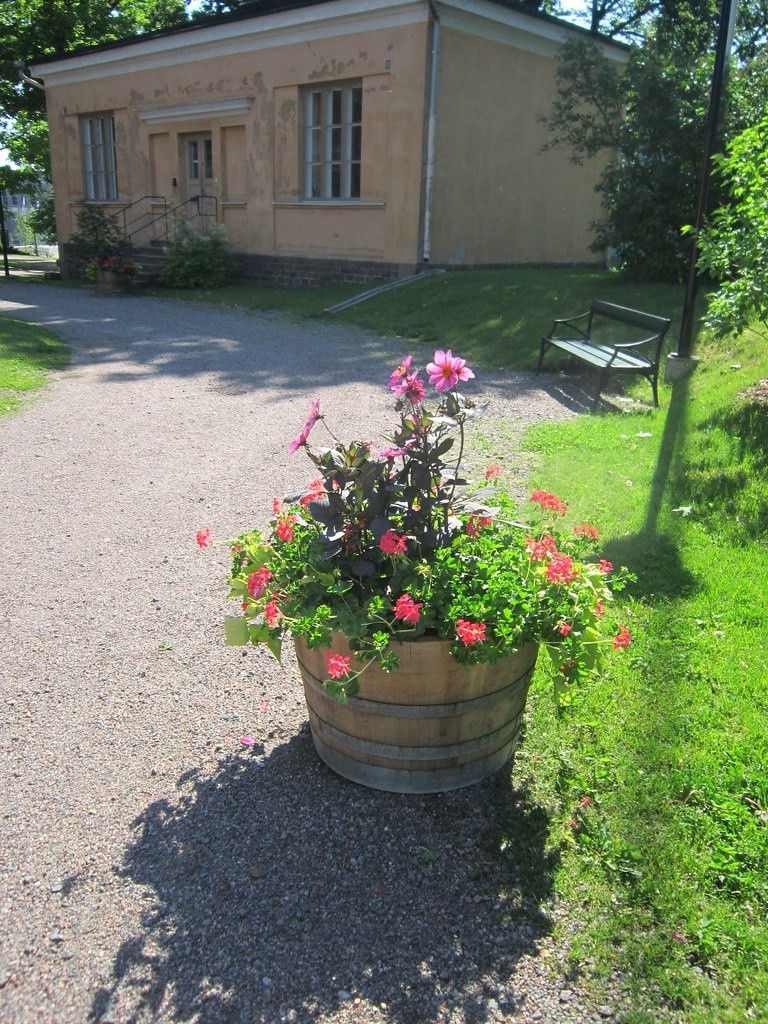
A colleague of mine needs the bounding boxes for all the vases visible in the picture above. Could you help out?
[285,511,549,793]
[95,270,131,295]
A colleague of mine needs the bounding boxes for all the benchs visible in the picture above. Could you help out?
[536,297,670,414]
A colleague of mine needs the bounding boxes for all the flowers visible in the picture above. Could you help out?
[86,234,139,283]
[197,351,638,707]
